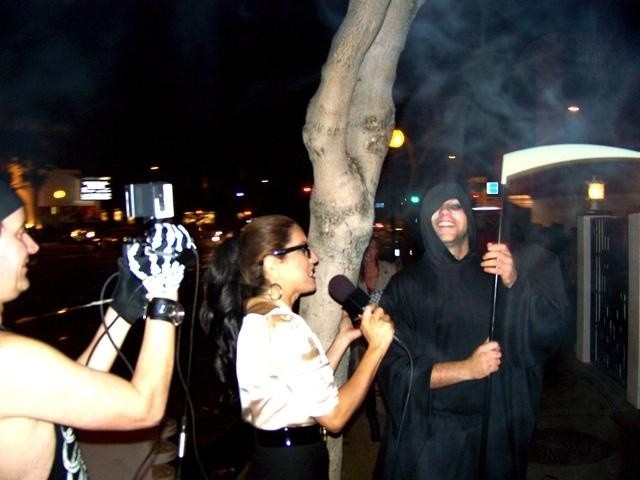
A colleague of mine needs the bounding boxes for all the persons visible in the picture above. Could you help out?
[371,182,574,478]
[355,233,396,441]
[1,179,192,480]
[198,213,399,480]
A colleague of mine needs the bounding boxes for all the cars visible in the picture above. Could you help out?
[373,211,403,255]
[194,208,262,271]
[69,223,131,251]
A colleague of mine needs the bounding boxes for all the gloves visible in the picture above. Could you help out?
[113,223,192,324]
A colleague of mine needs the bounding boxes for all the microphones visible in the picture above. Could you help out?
[329,276,405,351]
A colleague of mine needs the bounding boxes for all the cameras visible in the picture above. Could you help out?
[122,181,180,293]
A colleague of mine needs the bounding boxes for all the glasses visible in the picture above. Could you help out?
[274,242,312,259]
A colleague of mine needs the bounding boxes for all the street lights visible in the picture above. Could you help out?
[388,129,405,262]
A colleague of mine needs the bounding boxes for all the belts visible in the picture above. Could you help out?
[258,422,329,447]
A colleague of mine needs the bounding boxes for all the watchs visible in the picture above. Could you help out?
[141,297,187,326]
